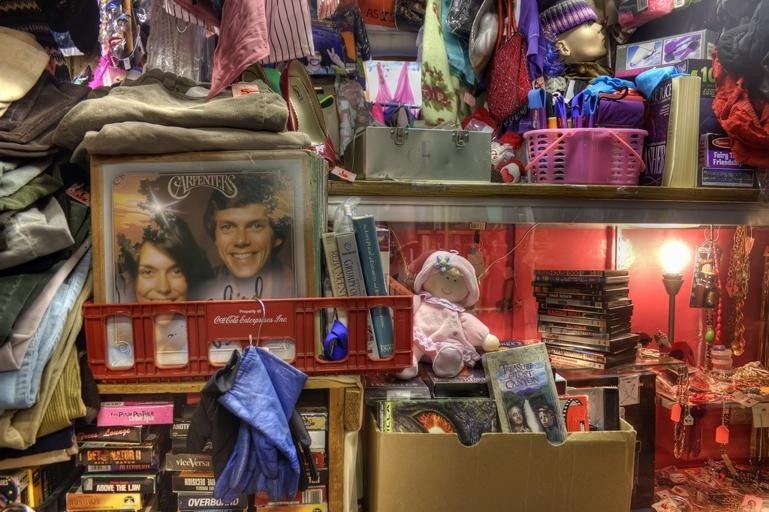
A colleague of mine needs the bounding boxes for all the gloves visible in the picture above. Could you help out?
[289,408,319,493]
[211,345,309,506]
[186,349,241,483]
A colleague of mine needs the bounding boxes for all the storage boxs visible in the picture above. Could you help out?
[368,401,636,512]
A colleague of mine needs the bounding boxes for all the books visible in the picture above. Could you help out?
[531,270,640,369]
[480,340,568,444]
[319,216,393,364]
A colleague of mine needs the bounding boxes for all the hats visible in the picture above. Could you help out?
[536,0,599,37]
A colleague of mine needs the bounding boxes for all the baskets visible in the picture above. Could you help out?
[522,126,651,186]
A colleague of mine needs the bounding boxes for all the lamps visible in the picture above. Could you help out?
[658,238,696,346]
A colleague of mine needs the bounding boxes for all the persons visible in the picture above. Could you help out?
[540,1,612,83]
[389,251,499,379]
[507,403,560,445]
[118,174,296,301]
